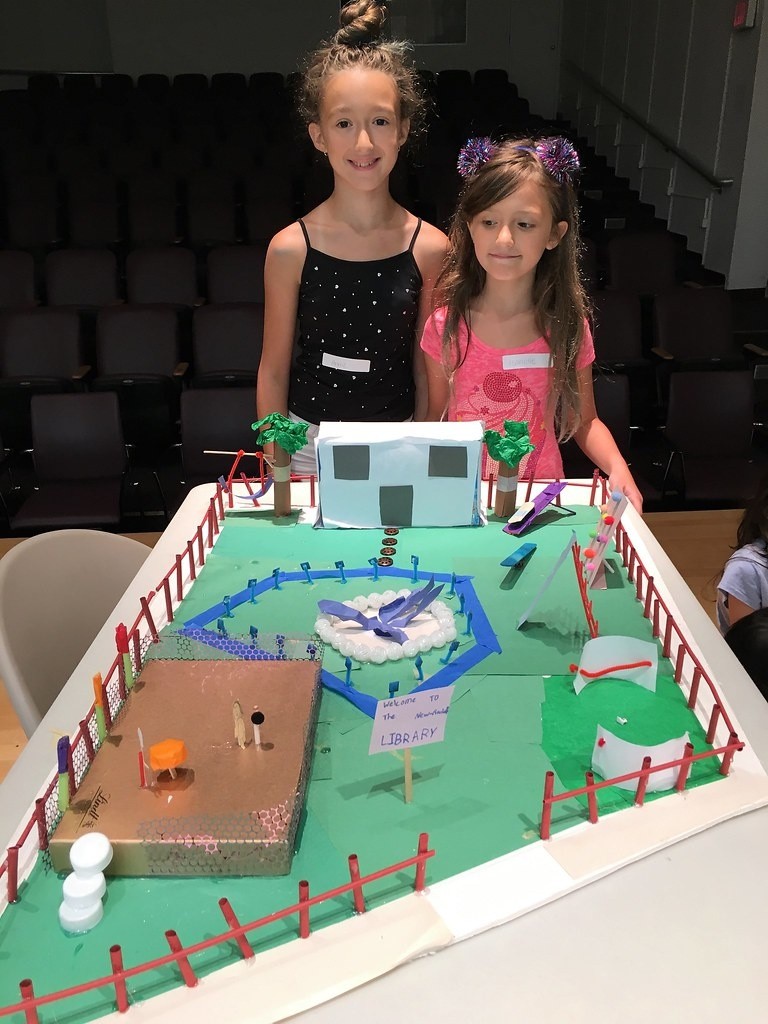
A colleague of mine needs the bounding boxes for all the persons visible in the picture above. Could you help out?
[255,0,451,484]
[420,136,643,516]
[702,483,768,624]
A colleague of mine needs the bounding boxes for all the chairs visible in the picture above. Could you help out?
[657,371,768,500]
[561,374,663,503]
[0,529,153,741]
[153,386,263,522]
[0,391,144,530]
[0,69,768,429]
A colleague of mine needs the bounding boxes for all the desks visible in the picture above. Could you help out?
[0,482,767,1024]
